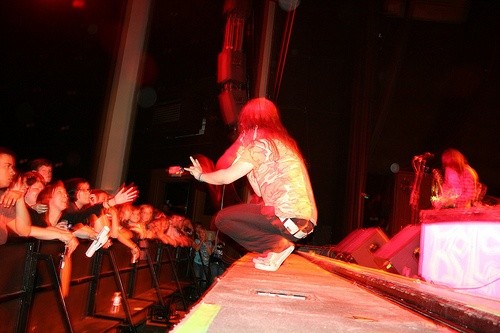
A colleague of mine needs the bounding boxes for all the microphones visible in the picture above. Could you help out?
[168,166,191,177]
[414,152,431,160]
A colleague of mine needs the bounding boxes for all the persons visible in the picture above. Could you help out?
[183,98,317,270]
[0,148,227,333]
[432,149,478,209]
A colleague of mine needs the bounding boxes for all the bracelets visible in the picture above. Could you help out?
[107,200,111,207]
[199,173,203,181]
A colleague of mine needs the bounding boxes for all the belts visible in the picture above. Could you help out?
[278,216,313,239]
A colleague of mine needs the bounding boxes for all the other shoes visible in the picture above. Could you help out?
[253,245,295,271]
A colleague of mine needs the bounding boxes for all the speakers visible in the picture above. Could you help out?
[331,227,390,268]
[373,224,421,278]
